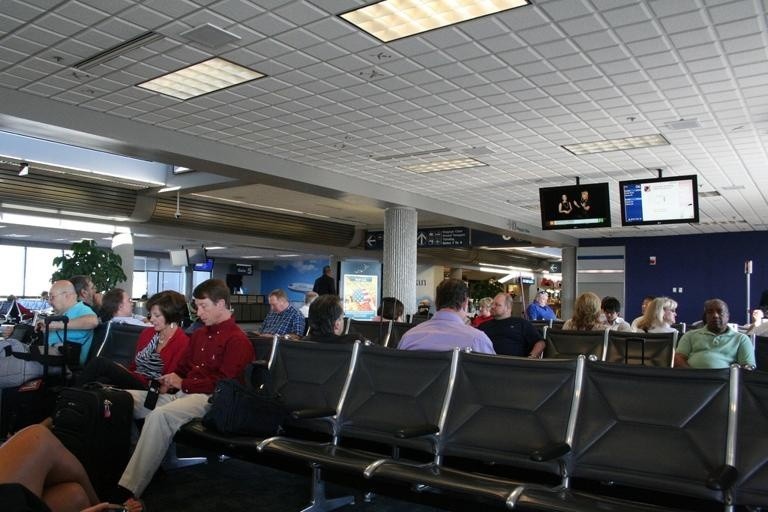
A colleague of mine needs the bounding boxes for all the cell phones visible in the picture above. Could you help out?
[144,376,179,411]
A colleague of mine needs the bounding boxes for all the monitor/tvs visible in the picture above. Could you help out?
[193,257,215,272]
[619,174,699,227]
[235,264,253,275]
[169,249,189,267]
[187,248,207,264]
[539,182,611,230]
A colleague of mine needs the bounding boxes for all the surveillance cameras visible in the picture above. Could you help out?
[175,212,182,219]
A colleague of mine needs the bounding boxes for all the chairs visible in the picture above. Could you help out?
[0,298,51,329]
[359,346,585,512]
[72,312,767,396]
[704,359,767,512]
[252,338,460,510]
[503,354,743,512]
[204,334,361,511]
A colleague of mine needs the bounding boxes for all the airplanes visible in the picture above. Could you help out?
[287,282,314,294]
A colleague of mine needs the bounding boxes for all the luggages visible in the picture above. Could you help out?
[3,315,134,502]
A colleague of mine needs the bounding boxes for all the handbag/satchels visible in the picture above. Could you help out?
[201,379,289,435]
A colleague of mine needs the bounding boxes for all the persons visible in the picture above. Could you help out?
[673,299,767,369]
[558,193,573,219]
[573,190,592,218]
[299,291,320,318]
[561,292,679,333]
[477,291,547,358]
[374,297,404,322]
[397,278,494,354]
[527,292,557,321]
[253,288,306,340]
[472,297,494,327]
[308,294,355,344]
[313,266,336,296]
[0,274,251,510]
